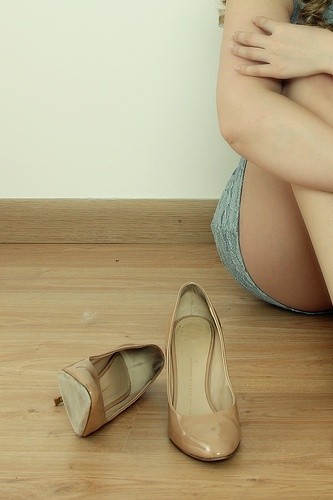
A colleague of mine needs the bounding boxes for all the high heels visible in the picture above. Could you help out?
[167,280,242,466]
[56,340,165,436]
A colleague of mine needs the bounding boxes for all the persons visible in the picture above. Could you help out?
[209,0,333,315]
